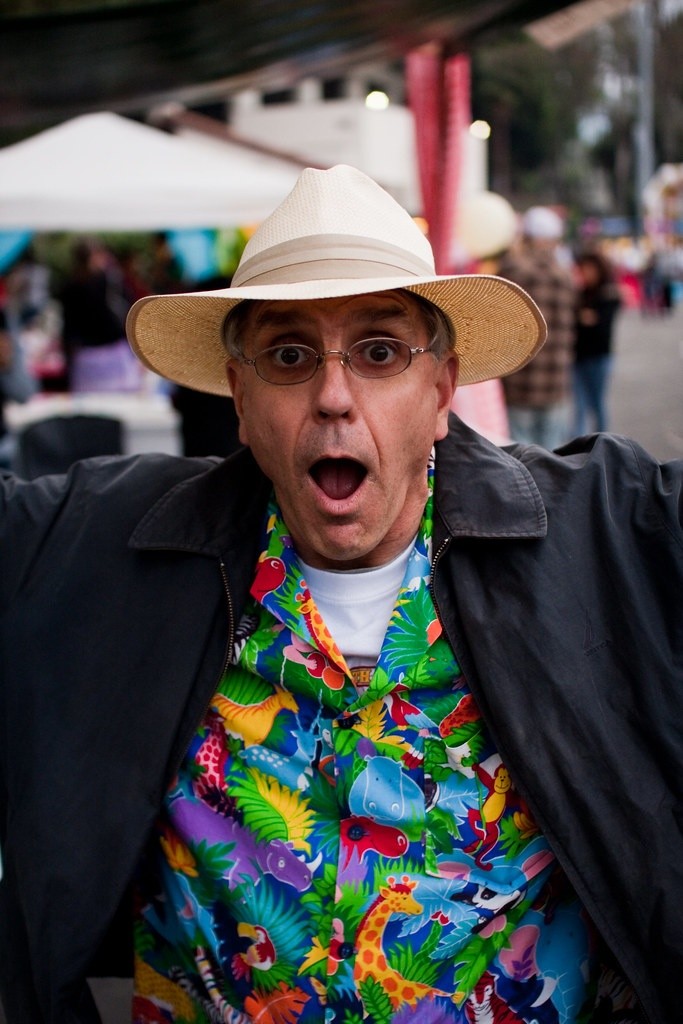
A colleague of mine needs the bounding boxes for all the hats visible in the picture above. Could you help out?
[524,205,564,240]
[125,165,547,398]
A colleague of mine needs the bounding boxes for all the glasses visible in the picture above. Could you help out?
[232,338,432,385]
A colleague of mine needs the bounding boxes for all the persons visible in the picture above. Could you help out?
[1,165,683,1023]
[0,206,683,447]
